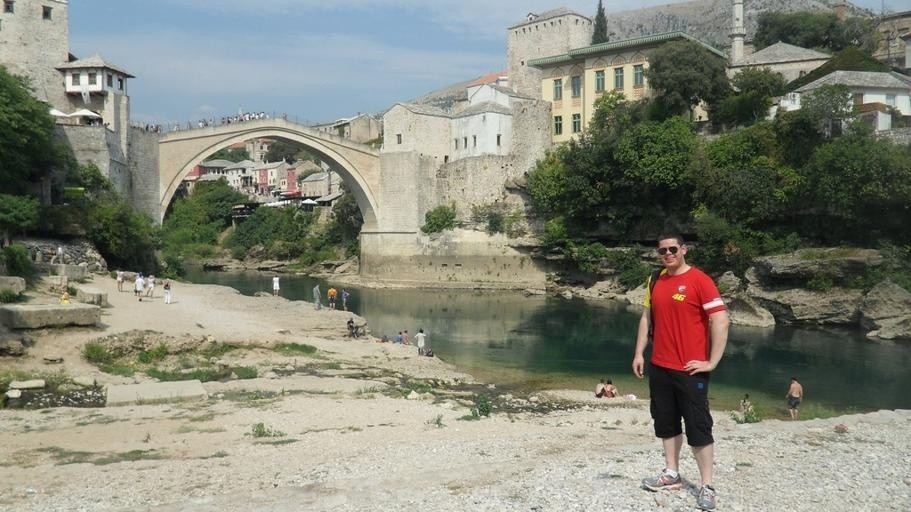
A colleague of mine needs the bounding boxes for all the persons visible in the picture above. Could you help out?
[785,376,804,421]
[146,274,157,298]
[401,329,411,346]
[116,268,124,292]
[394,331,402,344]
[413,327,427,355]
[738,393,752,414]
[271,273,280,297]
[56,243,65,265]
[101,111,287,134]
[162,277,174,304]
[327,285,337,311]
[312,284,322,311]
[631,232,730,511]
[341,288,350,311]
[347,317,358,338]
[133,271,143,297]
[58,292,72,305]
[135,275,145,302]
[603,379,616,398]
[593,378,606,398]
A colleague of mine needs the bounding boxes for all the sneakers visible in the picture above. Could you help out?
[642,470,682,491]
[696,485,716,511]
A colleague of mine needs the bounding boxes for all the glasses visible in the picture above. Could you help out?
[658,247,678,255]
